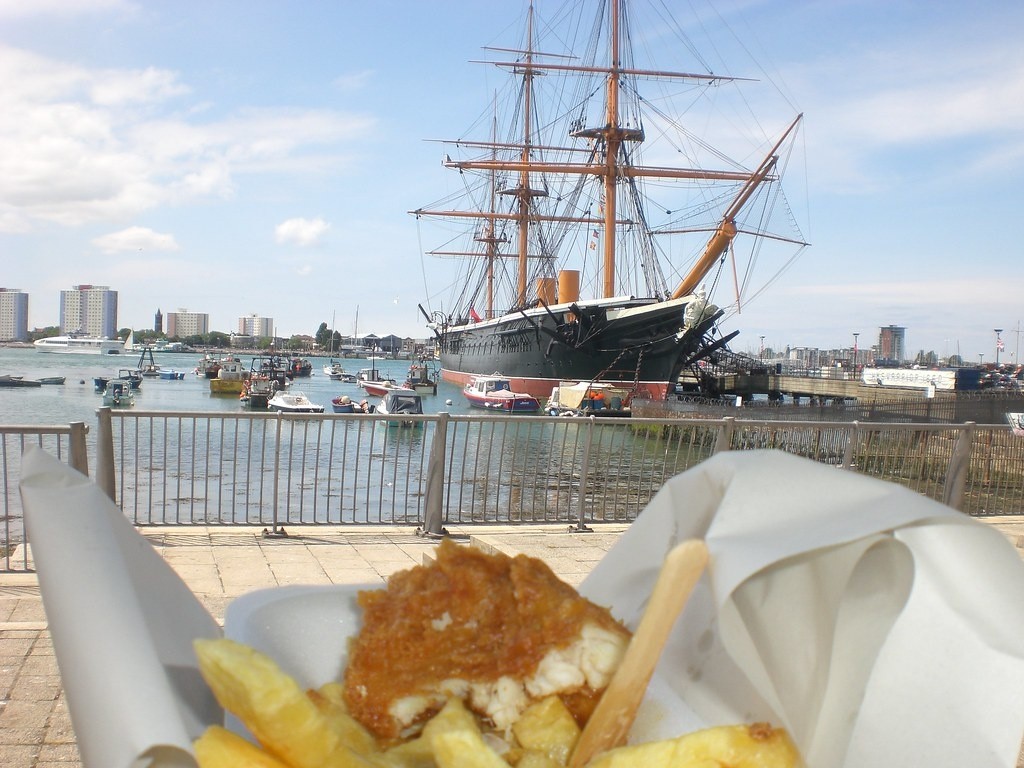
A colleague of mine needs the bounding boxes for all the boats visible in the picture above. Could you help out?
[402,364,437,396]
[194,351,312,408]
[102,379,136,408]
[544,381,632,417]
[359,379,415,398]
[0,375,42,388]
[376,388,424,428]
[267,391,325,414]
[35,376,66,384]
[333,396,375,414]
[463,371,542,412]
[34,334,126,355]
[94,342,185,390]
[329,371,359,384]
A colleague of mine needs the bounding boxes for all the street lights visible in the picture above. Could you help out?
[760,335,765,366]
[994,327,1005,371]
[978,352,985,366]
[853,331,859,380]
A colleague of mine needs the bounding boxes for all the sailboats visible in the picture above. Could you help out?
[405,0,812,416]
[354,341,397,387]
[324,309,347,375]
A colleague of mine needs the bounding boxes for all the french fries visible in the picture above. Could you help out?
[190,636,800,767]
